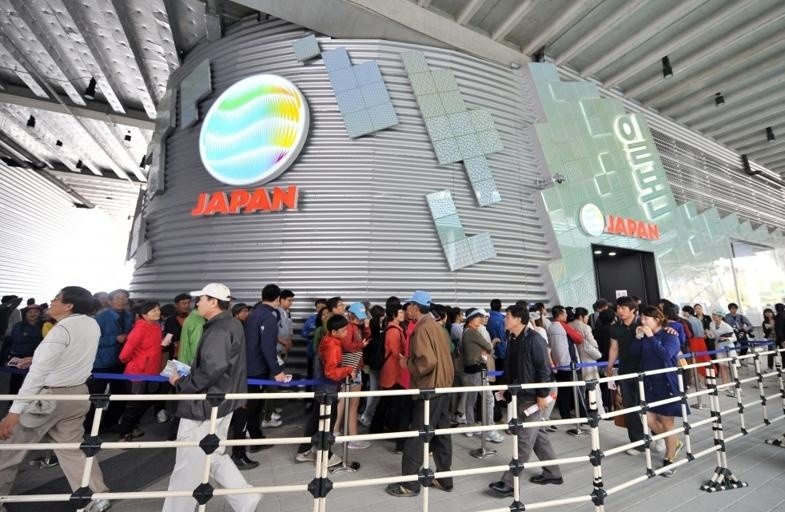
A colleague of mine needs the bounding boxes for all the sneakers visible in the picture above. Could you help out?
[85,498,110,512]
[429,478,452,492]
[261,419,282,427]
[485,430,503,442]
[662,460,676,477]
[333,433,344,444]
[271,412,281,419]
[157,410,168,423]
[296,450,317,462]
[655,437,667,452]
[465,430,481,437]
[238,493,263,512]
[132,429,144,437]
[348,440,371,450]
[120,435,130,450]
[386,483,419,497]
[670,439,684,459]
[327,454,343,467]
[626,448,640,455]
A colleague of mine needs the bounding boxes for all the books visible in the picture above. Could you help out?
[161,359,191,379]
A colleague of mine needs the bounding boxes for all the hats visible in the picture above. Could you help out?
[466,309,483,316]
[190,283,231,302]
[404,291,431,306]
[348,303,368,319]
[479,309,490,317]
[712,308,725,317]
[18,388,56,428]
[529,312,540,321]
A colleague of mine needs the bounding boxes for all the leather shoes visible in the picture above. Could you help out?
[530,476,563,485]
[488,480,514,496]
[249,445,273,453]
[231,454,259,470]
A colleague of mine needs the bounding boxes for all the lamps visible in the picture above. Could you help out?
[663,55,673,79]
[715,96,725,106]
[25,114,38,130]
[84,78,95,99]
[766,126,775,141]
[139,154,149,170]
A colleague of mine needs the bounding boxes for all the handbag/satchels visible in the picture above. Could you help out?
[338,352,364,371]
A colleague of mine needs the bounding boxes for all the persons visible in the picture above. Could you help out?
[165,293,192,421]
[296,314,356,467]
[359,305,386,427]
[393,320,417,454]
[490,306,563,494]
[386,297,400,307]
[693,304,721,378]
[39,303,57,324]
[548,305,586,417]
[657,299,667,311]
[595,300,617,410]
[0,295,23,350]
[313,306,333,353]
[477,309,507,423]
[629,305,691,467]
[449,306,468,424]
[128,298,143,323]
[90,299,101,317]
[775,303,785,373]
[41,309,57,337]
[178,297,208,367]
[21,299,37,321]
[94,292,110,315]
[371,303,405,433]
[516,301,558,431]
[300,299,327,392]
[230,284,285,470]
[163,284,264,512]
[724,303,755,367]
[462,308,506,444]
[7,305,43,407]
[704,310,741,398]
[486,300,508,408]
[119,302,163,449]
[663,303,695,393]
[607,296,680,456]
[83,289,134,433]
[333,302,372,450]
[588,302,602,336]
[763,309,779,373]
[232,302,249,320]
[430,302,452,331]
[386,291,455,497]
[682,306,717,378]
[158,304,174,423]
[0,287,111,512]
[328,297,346,314]
[260,289,294,427]
[566,308,607,414]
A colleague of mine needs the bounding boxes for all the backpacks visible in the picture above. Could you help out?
[314,354,338,402]
[363,325,402,370]
